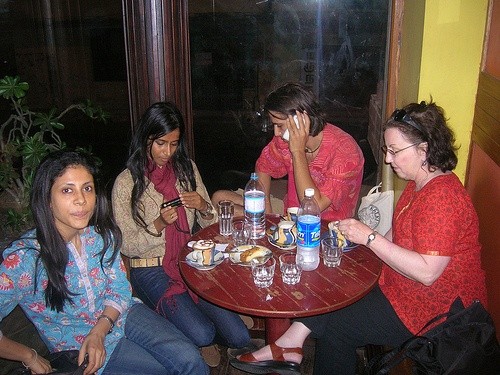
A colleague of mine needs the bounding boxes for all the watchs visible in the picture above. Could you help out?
[365,230,378,248]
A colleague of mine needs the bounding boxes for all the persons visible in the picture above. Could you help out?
[229,95,490,375]
[110,100,255,368]
[212,78,365,222]
[1,145,211,375]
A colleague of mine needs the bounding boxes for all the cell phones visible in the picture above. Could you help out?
[284,115,306,142]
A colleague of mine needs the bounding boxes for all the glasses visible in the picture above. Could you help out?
[381,141,422,155]
[390,109,422,138]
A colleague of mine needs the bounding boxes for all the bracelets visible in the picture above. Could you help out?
[144,225,163,238]
[97,314,115,335]
[200,201,212,217]
[21,348,38,369]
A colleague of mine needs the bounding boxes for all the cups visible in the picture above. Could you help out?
[321,237,344,267]
[287,207,302,225]
[327,220,354,248]
[279,251,304,285]
[230,220,252,246]
[217,200,234,237]
[251,256,276,288]
[192,245,215,266]
[277,220,297,245]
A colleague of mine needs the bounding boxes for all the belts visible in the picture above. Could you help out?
[129,257,163,268]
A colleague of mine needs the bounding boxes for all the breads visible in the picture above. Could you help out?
[240,246,266,262]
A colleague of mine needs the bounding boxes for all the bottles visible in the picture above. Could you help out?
[297,187,321,270]
[244,173,266,238]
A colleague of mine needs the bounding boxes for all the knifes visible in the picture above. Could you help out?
[221,250,244,253]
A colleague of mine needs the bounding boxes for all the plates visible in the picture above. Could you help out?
[230,245,272,265]
[321,231,359,252]
[185,250,224,270]
[280,216,287,221]
[267,235,298,251]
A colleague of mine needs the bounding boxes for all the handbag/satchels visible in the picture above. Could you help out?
[358,181,395,236]
[365,296,500,375]
[6,350,95,375]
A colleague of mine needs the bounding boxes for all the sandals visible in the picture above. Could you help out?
[230,342,304,375]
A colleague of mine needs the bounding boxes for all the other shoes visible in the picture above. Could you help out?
[201,344,221,368]
[239,314,254,330]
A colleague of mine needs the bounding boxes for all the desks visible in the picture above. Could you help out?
[178,215,382,347]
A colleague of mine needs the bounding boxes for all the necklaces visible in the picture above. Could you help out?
[304,139,324,154]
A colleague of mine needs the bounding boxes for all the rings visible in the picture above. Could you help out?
[171,214,174,219]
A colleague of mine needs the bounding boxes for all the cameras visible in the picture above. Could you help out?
[162,197,183,209]
